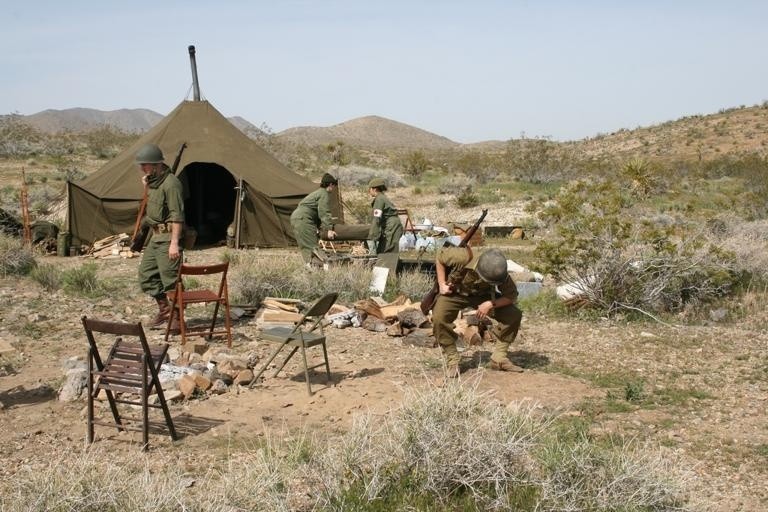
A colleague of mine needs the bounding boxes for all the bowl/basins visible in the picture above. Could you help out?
[413,224,433,230]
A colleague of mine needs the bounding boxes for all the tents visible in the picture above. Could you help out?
[67,100,344,257]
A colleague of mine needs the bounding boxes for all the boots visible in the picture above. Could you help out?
[490,339,524,373]
[442,343,463,378]
[169,307,186,335]
[145,293,171,327]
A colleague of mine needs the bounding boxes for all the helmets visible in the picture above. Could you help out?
[474,250,508,285]
[135,143,165,164]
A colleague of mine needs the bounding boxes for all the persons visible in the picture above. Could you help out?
[291,173,337,263]
[368,179,403,254]
[136,144,186,335]
[433,248,523,377]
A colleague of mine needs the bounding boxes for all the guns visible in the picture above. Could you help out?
[419,209,487,315]
[130,142,186,252]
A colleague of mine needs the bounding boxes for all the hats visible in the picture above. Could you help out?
[368,178,385,188]
[321,172,338,184]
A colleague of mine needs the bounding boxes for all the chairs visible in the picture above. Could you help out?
[81,314,179,452]
[247,292,340,397]
[394,209,422,241]
[162,260,233,348]
